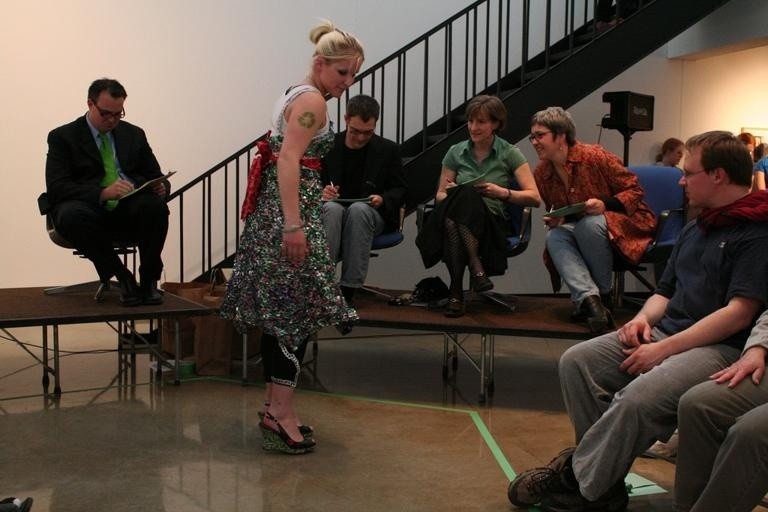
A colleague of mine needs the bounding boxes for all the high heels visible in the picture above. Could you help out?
[568,291,615,322]
[576,295,617,330]
[256,411,314,437]
[258,422,317,454]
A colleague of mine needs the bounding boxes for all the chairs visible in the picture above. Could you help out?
[416,174,531,312]
[38,193,137,302]
[568,166,687,323]
[336,205,405,305]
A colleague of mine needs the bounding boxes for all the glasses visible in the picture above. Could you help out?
[94,105,125,120]
[349,122,374,138]
[682,170,705,176]
[529,130,552,144]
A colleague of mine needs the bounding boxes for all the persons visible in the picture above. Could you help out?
[219,19,366,455]
[655,138,686,168]
[435,94,542,318]
[671,308,767,512]
[736,132,767,194]
[529,107,659,336]
[45,79,171,307]
[321,95,408,312]
[506,131,767,512]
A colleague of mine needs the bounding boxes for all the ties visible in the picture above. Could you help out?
[98,132,120,211]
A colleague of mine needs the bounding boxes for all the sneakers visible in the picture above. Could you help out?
[508,447,576,509]
[641,428,679,458]
[541,477,631,512]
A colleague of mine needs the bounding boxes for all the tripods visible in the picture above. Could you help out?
[612,136,657,304]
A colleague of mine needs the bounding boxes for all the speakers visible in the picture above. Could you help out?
[601,91,655,136]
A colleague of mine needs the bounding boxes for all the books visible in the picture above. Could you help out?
[331,197,371,205]
[118,170,177,200]
[543,202,586,217]
[446,173,487,195]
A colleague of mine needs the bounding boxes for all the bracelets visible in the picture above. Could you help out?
[505,189,513,202]
[281,225,301,234]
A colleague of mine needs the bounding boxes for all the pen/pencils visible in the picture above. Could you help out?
[330,181,340,197]
[447,179,452,183]
[118,171,127,180]
[544,204,554,232]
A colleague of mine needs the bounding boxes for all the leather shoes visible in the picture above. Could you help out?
[443,297,466,317]
[140,281,165,305]
[118,269,142,307]
[471,271,493,292]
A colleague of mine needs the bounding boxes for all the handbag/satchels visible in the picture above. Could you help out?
[413,276,448,302]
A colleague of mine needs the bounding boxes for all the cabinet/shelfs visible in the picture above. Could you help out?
[0,282,212,407]
[312,285,654,406]
